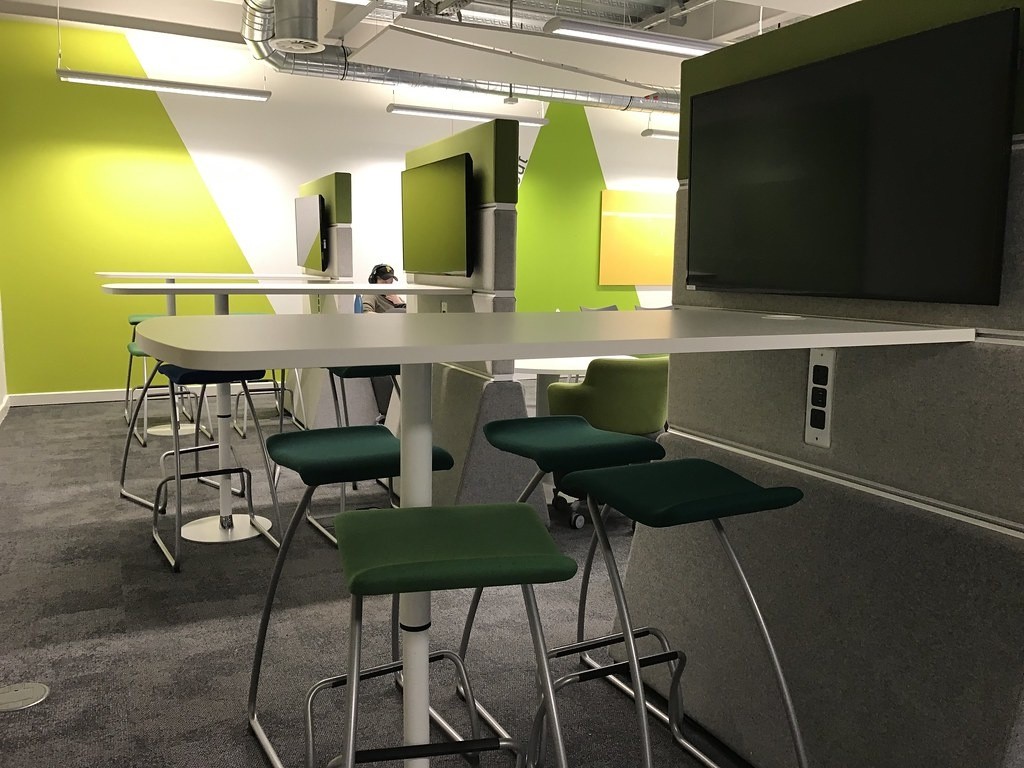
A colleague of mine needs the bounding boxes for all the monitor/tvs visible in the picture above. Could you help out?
[401,153,475,277]
[294,194,329,272]
[685,7,1021,308]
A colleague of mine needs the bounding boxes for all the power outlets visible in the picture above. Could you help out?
[804,348,835,447]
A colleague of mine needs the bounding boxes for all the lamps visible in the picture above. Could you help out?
[641,111,679,140]
[56,0,272,102]
[386,87,549,127]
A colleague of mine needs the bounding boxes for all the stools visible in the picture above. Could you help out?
[127,342,214,448]
[151,364,283,570]
[247,426,480,768]
[333,502,578,767]
[454,413,666,768]
[561,459,809,768]
[123,315,194,426]
[307,363,401,544]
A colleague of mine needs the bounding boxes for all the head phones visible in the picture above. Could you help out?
[368,265,394,284]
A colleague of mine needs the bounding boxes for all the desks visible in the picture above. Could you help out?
[135,304,979,768]
[102,283,472,543]
[98,272,332,436]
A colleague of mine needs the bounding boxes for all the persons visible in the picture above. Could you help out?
[359,264,407,314]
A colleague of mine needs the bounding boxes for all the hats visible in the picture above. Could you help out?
[372,265,398,281]
